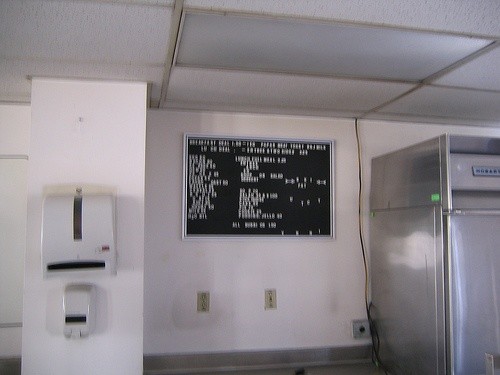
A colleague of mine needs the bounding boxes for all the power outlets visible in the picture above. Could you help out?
[197,291,208,311]
[265,289,275,309]
[352,321,369,338]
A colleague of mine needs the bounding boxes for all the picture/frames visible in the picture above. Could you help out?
[182,132,335,239]
